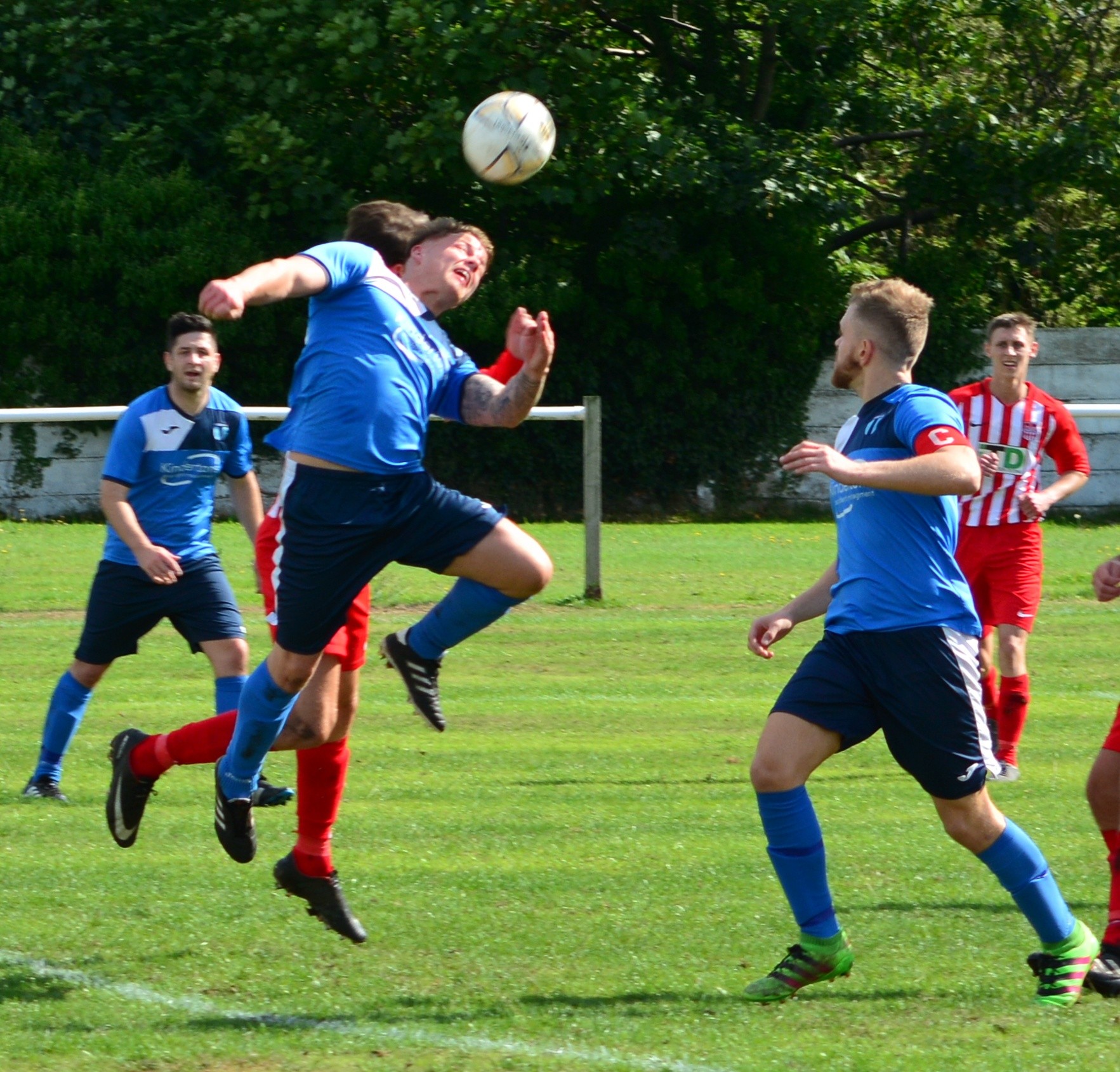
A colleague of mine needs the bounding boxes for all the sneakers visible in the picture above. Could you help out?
[986,719,997,755]
[252,775,296,807]
[215,758,256,864]
[107,727,161,848]
[275,854,367,945]
[745,929,855,1001]
[376,623,445,733]
[986,762,1018,781]
[1025,919,1100,1008]
[23,780,67,803]
[1083,954,1120,999]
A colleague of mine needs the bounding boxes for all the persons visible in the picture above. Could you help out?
[104,197,539,944]
[1027,552,1120,1000]
[743,274,1102,1009]
[19,312,295,811]
[945,310,1093,784]
[198,214,555,863]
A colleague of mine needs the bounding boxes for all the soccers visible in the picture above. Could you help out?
[462,90,557,186]
